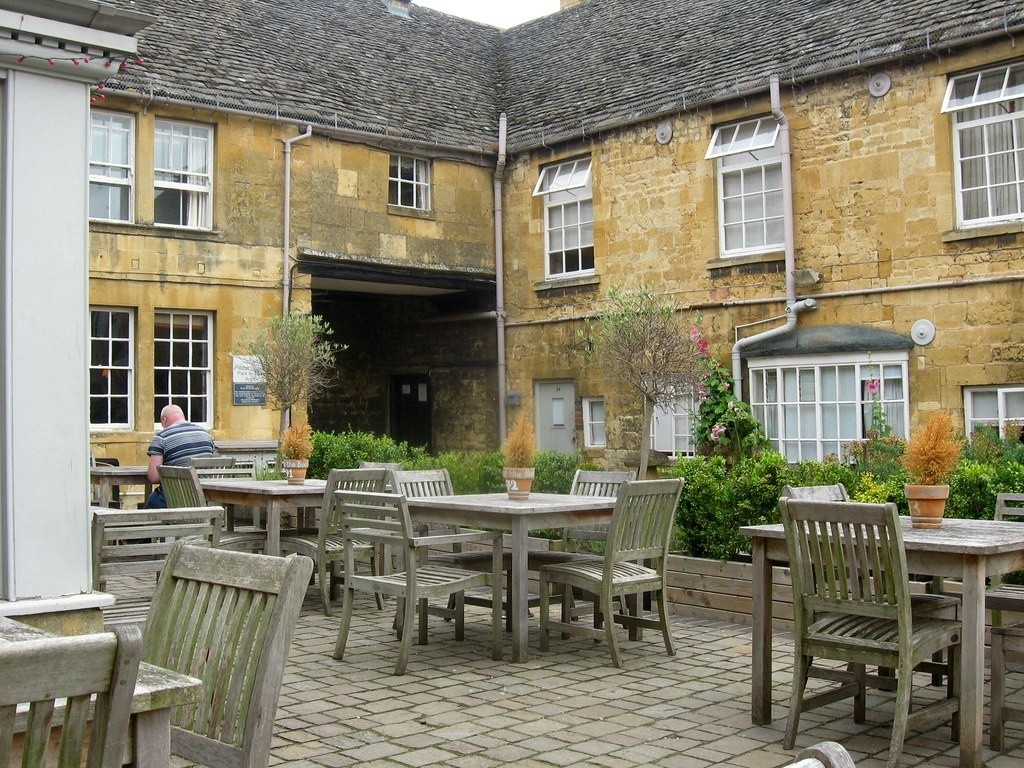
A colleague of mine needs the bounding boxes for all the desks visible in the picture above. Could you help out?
[407,491,650,665]
[0,614,205,768]
[89,465,154,512]
[737,515,1024,768]
[201,477,393,557]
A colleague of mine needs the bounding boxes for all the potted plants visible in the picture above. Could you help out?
[498,404,538,501]
[904,404,969,529]
[279,415,314,484]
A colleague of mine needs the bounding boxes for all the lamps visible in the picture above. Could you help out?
[790,269,822,288]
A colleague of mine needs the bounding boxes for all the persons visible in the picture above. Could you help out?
[143,404,215,509]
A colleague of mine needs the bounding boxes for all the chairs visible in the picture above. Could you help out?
[0,456,1024,768]
